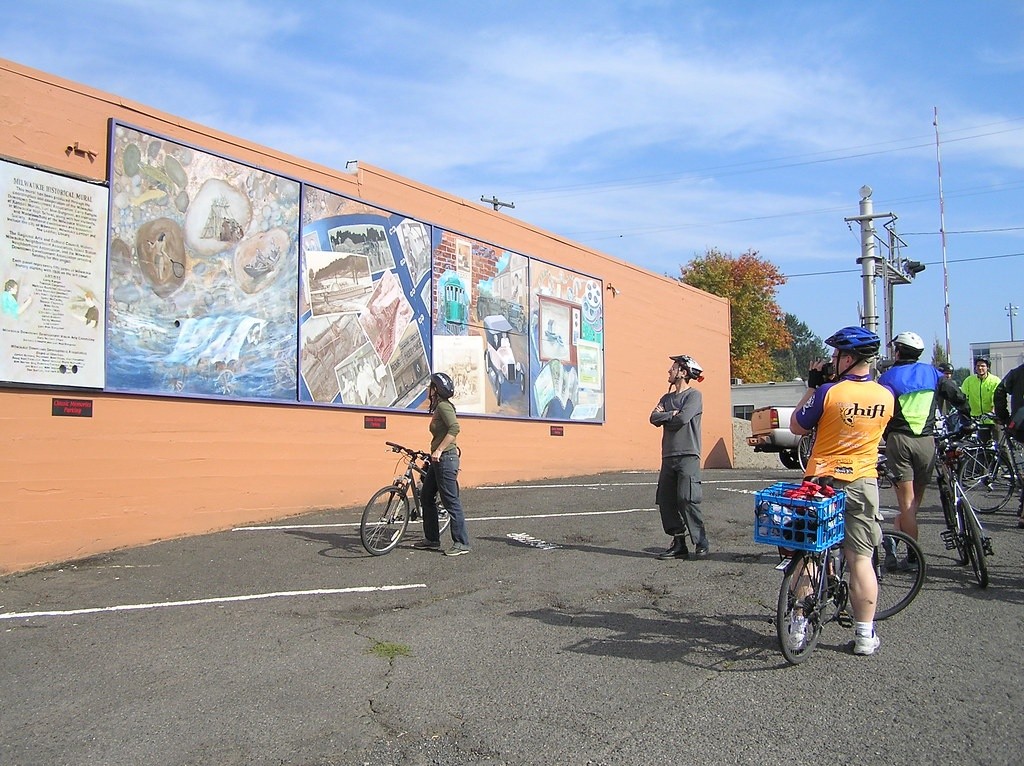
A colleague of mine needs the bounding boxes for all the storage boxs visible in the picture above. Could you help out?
[754,482,847,551]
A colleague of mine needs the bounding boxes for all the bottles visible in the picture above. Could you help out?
[822,363,835,376]
[417,481,423,505]
[1014,451,1024,478]
[831,545,840,578]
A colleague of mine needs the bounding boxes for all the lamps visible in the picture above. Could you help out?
[606,283,620,295]
[67,142,99,157]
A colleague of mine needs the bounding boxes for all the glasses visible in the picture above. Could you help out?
[831,353,855,360]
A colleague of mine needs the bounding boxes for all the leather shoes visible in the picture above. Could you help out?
[694,538,710,558]
[657,535,690,559]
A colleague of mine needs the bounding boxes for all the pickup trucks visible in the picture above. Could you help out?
[746,403,816,469]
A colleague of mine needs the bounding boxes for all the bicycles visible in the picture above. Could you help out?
[774,474,926,665]
[873,413,1024,514]
[361,442,459,557]
[926,426,994,588]
[797,423,817,472]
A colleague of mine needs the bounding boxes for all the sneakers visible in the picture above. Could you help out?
[444,546,470,556]
[414,542,439,550]
[787,619,808,650]
[854,629,881,655]
[897,556,919,571]
[883,536,897,571]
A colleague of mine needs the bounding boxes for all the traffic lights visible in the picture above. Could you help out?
[904,262,925,278]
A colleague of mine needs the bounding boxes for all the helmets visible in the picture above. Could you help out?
[429,372,454,398]
[975,354,991,368]
[824,326,881,356]
[887,332,924,353]
[668,354,704,380]
[938,363,954,373]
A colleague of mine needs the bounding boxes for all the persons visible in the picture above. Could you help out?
[938,362,957,417]
[992,361,1024,532]
[648,354,711,560]
[782,325,896,656]
[412,371,472,557]
[878,331,973,571]
[959,354,1009,491]
